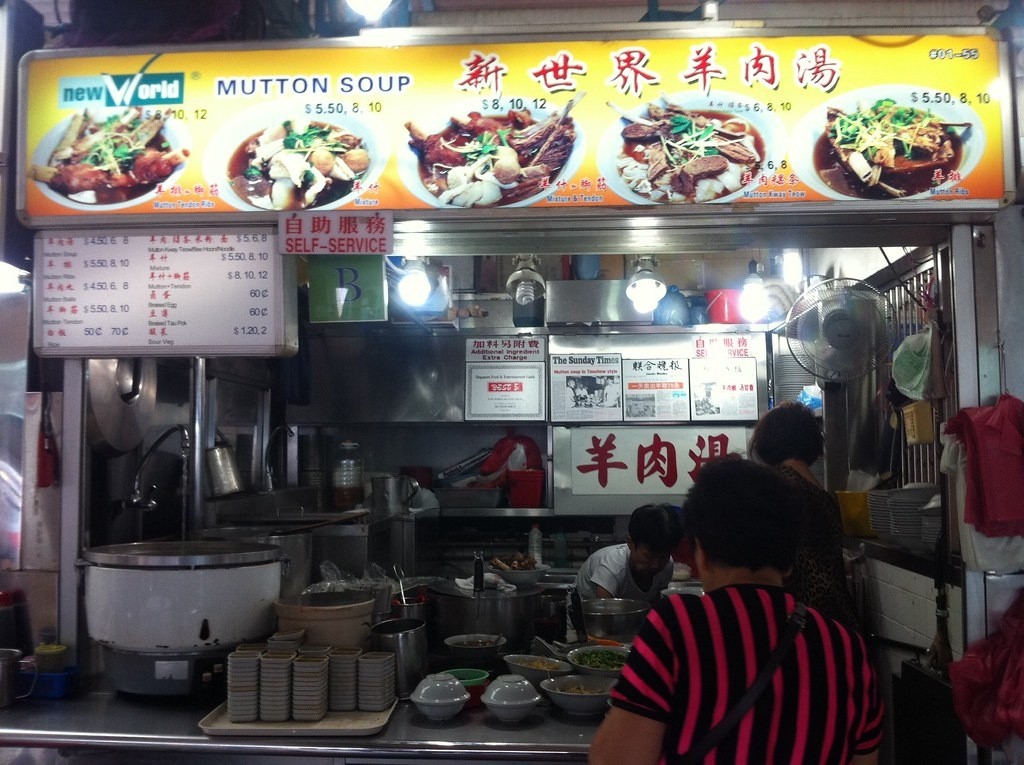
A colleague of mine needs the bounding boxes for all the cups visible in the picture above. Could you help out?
[0,648,38,709]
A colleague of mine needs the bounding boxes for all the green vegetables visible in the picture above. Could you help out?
[244,120,340,181]
[461,128,525,163]
[827,99,932,159]
[670,117,719,165]
[572,649,626,670]
[83,114,171,169]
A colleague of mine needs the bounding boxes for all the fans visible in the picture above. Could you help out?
[785,278,897,382]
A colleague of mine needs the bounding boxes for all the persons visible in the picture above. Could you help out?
[567,504,684,638]
[749,403,859,638]
[566,376,619,407]
[588,456,885,765]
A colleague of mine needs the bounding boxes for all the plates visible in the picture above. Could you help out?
[30,110,193,212]
[201,111,386,213]
[866,487,944,554]
[597,91,785,207]
[226,626,396,723]
[395,97,586,210]
[785,83,985,200]
[566,645,631,671]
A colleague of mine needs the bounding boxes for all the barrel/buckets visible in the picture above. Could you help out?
[704,288,742,324]
[508,470,543,508]
[370,475,410,516]
[200,442,246,500]
[369,618,433,701]
[835,488,875,538]
[274,591,377,654]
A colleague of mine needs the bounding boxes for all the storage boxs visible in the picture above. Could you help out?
[434,470,545,508]
[836,491,877,539]
[16,667,82,699]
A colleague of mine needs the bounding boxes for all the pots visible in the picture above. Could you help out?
[69,538,286,700]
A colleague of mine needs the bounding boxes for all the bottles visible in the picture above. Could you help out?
[528,523,543,564]
[332,440,366,511]
[687,296,710,325]
[653,285,692,327]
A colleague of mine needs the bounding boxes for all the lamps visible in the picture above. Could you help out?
[507,253,545,306]
[625,253,667,315]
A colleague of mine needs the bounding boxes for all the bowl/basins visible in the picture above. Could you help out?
[480,674,542,723]
[566,598,652,643]
[488,564,551,587]
[503,654,573,685]
[440,670,489,709]
[539,674,619,716]
[410,673,472,720]
[444,634,507,658]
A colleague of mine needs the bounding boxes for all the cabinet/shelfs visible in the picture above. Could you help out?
[845,542,965,764]
